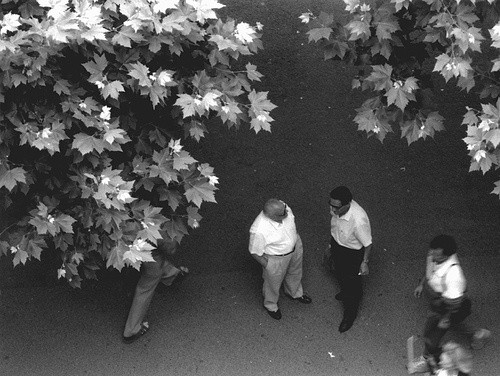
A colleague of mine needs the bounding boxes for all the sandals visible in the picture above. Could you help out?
[122,321,150,344]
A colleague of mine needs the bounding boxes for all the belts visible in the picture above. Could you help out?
[275,246,295,257]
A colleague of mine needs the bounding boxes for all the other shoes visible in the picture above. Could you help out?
[471,329,492,350]
[335,291,346,301]
[170,265,189,287]
[291,294,312,304]
[263,305,282,320]
[338,314,354,333]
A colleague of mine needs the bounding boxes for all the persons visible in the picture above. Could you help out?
[412,235,490,376]
[328,187,373,332]
[248,198,312,320]
[123,247,189,343]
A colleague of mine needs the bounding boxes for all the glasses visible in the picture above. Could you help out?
[278,202,286,216]
[328,200,345,209]
